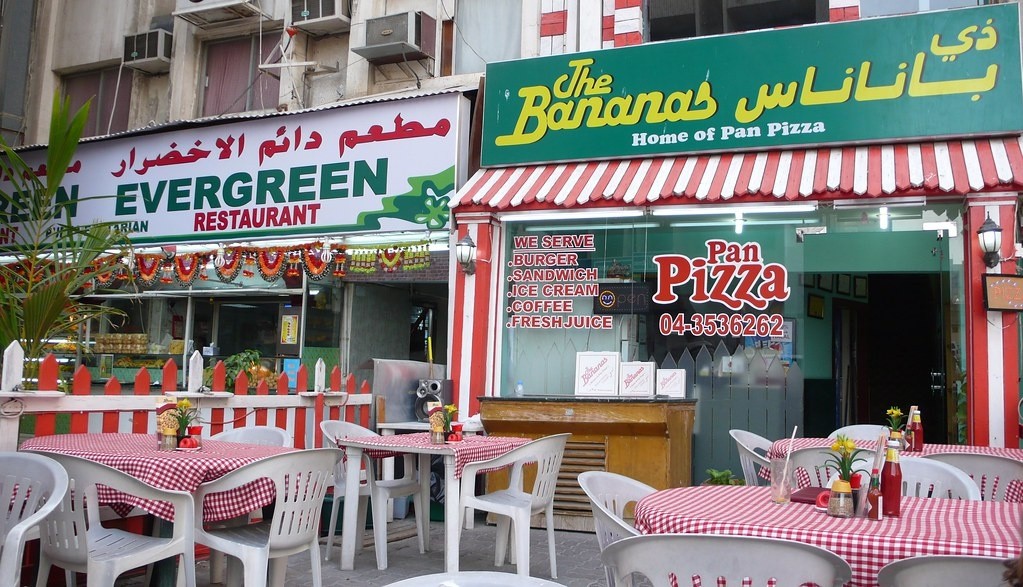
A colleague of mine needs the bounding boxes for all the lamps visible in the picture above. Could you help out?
[455,229,477,275]
[977,212,1004,268]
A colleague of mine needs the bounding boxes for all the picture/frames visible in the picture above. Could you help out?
[807,293,825,319]
[804,274,816,288]
[836,274,851,295]
[854,275,867,297]
[817,274,834,292]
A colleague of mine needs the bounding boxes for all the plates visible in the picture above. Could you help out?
[444,439,464,444]
[176,447,201,451]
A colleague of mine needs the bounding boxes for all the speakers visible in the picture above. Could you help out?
[415,379,453,423]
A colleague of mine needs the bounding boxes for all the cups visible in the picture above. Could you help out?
[770,458,793,504]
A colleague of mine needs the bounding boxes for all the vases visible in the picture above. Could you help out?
[850,488,859,514]
[444,432,455,441]
[176,435,191,447]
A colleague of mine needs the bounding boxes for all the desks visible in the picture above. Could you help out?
[19,433,309,587]
[635,486,1023,587]
[758,438,1023,502]
[335,432,534,572]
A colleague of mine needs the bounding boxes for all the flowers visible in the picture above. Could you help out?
[886,406,907,432]
[820,435,871,482]
[170,399,205,436]
[443,404,459,432]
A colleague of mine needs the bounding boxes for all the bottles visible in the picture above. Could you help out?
[187,426,202,447]
[432,426,444,445]
[905,406,923,452]
[867,469,883,521]
[881,441,902,517]
[826,480,854,518]
[890,432,902,450]
[452,424,462,441]
[162,426,177,452]
[514,381,524,398]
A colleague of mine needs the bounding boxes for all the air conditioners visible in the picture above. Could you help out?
[120,0,422,71]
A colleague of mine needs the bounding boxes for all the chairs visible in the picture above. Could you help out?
[0,420,1023,587]
